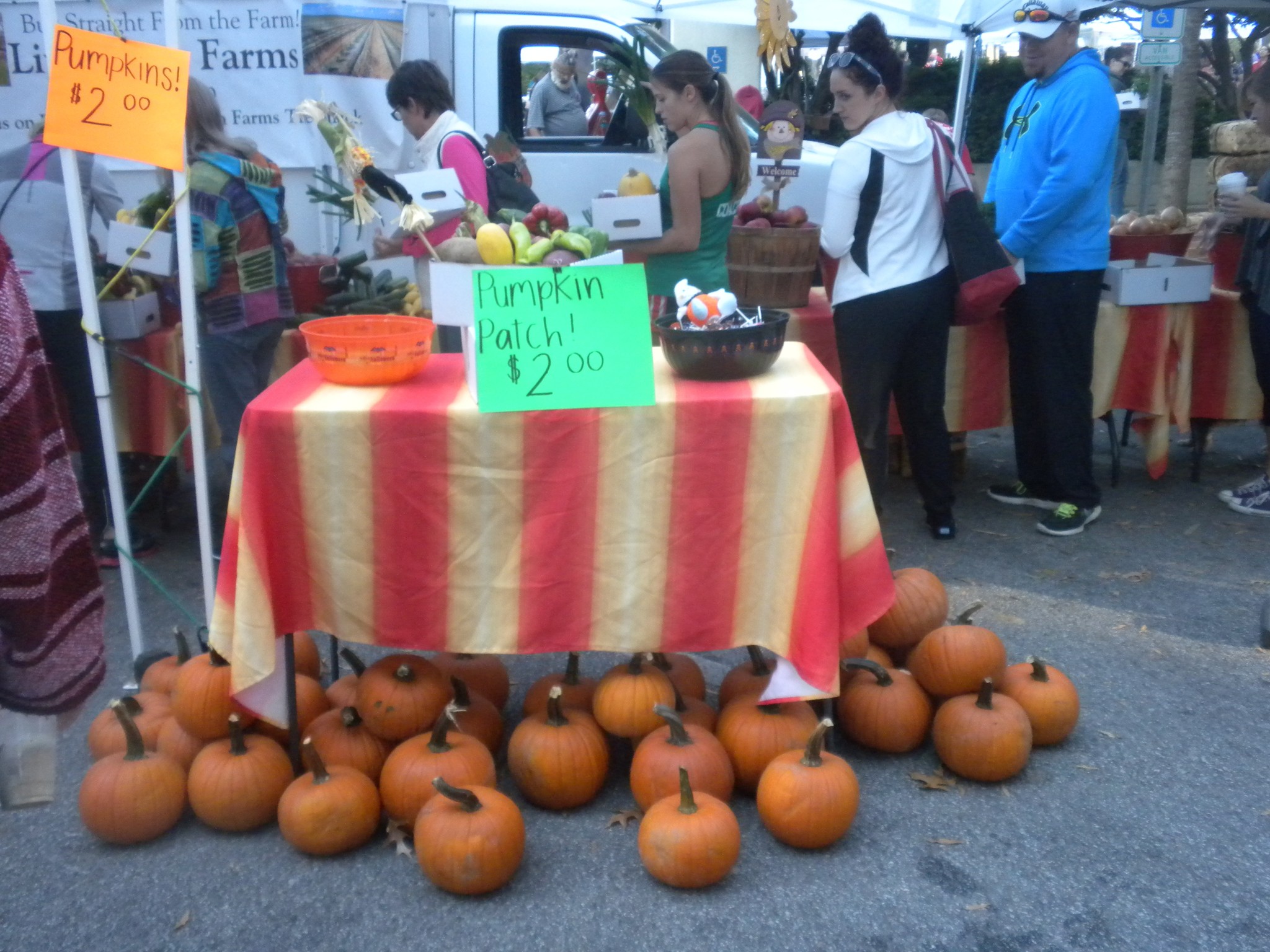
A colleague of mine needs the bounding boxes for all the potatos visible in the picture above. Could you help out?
[731,194,818,229]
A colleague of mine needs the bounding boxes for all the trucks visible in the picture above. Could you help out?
[3,0,850,282]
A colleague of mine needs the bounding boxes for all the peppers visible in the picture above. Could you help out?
[523,202,609,258]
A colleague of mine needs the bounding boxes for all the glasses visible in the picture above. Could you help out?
[1116,58,1133,71]
[826,52,883,85]
[1013,9,1072,25]
[390,100,408,122]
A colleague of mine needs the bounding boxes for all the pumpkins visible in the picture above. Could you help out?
[354,653,448,742]
[716,692,825,793]
[278,738,380,856]
[647,652,705,700]
[591,653,678,739]
[441,674,505,755]
[629,703,735,815]
[840,546,1004,751]
[932,677,1033,782]
[506,684,609,811]
[430,652,510,711]
[325,648,368,709]
[300,705,385,784]
[755,717,861,850]
[617,167,656,197]
[997,659,1080,746]
[79,624,328,846]
[414,776,525,894]
[672,684,718,733]
[380,713,495,834]
[637,765,741,887]
[719,645,778,704]
[523,651,597,714]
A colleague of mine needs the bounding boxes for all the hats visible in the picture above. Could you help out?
[1005,0,1080,39]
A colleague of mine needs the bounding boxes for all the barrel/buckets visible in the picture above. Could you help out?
[286,255,341,313]
[727,221,823,309]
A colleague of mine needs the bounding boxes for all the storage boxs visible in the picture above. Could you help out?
[106,219,178,278]
[357,167,468,262]
[592,187,663,242]
[97,291,162,340]
[1099,251,1214,305]
[430,247,623,354]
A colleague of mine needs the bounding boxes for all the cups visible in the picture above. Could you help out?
[1216,172,1248,224]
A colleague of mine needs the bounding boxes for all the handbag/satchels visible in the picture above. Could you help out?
[941,189,1022,327]
[436,129,541,227]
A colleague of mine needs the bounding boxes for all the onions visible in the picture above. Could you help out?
[1109,206,1184,236]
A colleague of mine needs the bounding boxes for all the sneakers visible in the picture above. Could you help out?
[1036,501,1103,537]
[985,479,1060,511]
[933,526,956,543]
[94,526,157,565]
[1218,470,1270,517]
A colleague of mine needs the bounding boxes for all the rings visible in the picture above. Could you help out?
[376,251,380,255]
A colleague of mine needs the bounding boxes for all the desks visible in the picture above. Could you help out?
[209,341,896,778]
[101,327,310,471]
[768,285,1265,490]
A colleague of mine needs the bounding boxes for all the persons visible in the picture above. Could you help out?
[0,232,111,731]
[980,0,1121,537]
[1252,44,1269,72]
[525,50,588,138]
[1196,62,1270,517]
[819,10,976,541]
[368,57,492,257]
[1230,50,1244,86]
[614,47,752,346]
[152,75,295,559]
[0,120,160,567]
[1101,45,1134,216]
[924,48,944,68]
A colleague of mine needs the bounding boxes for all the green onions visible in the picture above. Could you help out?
[586,32,668,154]
[306,170,377,221]
